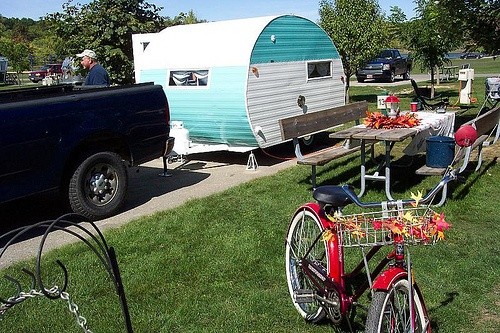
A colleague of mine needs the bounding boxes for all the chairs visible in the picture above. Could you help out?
[411,78,449,110]
[484,77,500,109]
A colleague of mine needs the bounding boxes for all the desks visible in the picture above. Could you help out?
[440,65,459,82]
[331,109,468,202]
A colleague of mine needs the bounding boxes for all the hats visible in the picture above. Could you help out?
[76,48,97,59]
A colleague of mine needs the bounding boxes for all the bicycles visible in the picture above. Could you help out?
[283,167,465,333]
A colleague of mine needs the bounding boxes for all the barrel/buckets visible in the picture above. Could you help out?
[425,136,455,167]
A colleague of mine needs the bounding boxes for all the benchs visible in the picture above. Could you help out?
[428,64,471,81]
[415,106,500,208]
[279,100,369,198]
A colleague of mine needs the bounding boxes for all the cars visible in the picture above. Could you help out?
[460,51,483,59]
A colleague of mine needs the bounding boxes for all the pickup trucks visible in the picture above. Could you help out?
[0,80,175,223]
[355,48,413,83]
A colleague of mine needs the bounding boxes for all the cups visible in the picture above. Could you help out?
[410,102,418,112]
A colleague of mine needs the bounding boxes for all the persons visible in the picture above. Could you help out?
[75,49,110,87]
[73,71,83,85]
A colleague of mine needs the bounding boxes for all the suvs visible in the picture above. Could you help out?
[28,64,63,83]
